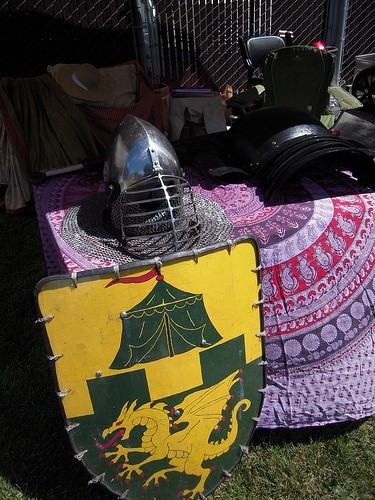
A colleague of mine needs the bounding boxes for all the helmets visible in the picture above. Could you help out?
[103,113,182,199]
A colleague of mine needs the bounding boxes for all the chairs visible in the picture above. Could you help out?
[227,44,363,136]
[238,31,295,89]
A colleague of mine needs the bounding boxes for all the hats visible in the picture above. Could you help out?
[55,62,113,101]
